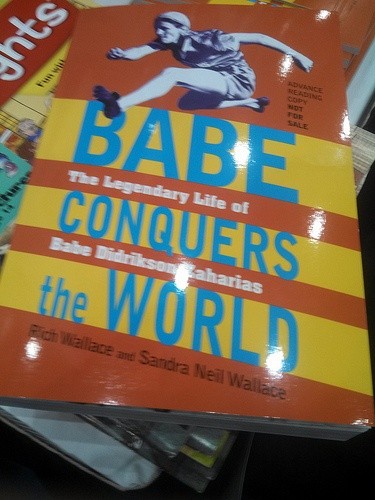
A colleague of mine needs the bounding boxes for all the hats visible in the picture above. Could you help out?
[157,12,190,30]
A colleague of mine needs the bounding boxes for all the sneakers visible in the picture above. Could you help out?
[253,96,269,112]
[93,85,121,118]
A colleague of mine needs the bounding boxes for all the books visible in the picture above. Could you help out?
[0,0,372,447]
[1,1,101,255]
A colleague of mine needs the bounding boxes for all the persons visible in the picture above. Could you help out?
[89,11,316,121]
